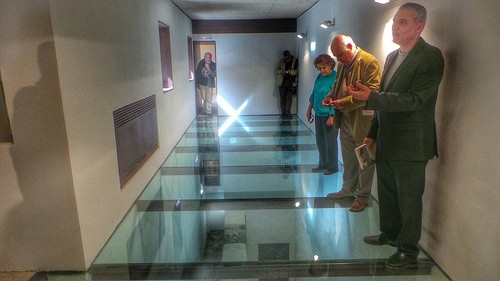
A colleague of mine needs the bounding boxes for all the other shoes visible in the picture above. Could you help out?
[323,170,337,175]
[312,166,321,170]
[327,188,352,198]
[281,114,293,120]
[349,199,369,212]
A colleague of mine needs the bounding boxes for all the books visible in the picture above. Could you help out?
[354,141,377,170]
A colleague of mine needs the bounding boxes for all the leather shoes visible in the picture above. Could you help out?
[386,250,415,269]
[363,234,398,246]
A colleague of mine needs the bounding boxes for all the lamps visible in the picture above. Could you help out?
[320,21,335,28]
[296,33,307,39]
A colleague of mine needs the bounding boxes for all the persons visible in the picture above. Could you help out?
[306,175,371,281]
[306,54,339,175]
[272,49,299,120]
[322,34,382,213]
[196,53,216,115]
[348,3,445,270]
[273,119,299,179]
